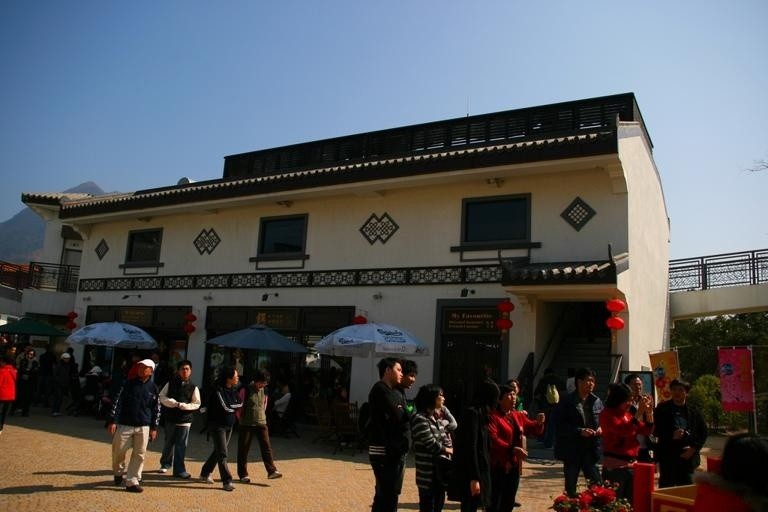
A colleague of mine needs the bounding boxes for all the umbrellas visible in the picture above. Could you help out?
[204,321,310,369]
[313,320,431,387]
[0,318,66,337]
[64,320,158,374]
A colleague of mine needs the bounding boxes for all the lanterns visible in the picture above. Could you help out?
[353,314,367,324]
[66,321,76,329]
[606,295,626,314]
[185,312,197,322]
[496,315,513,334]
[607,315,625,336]
[68,311,78,320]
[497,299,515,317]
[184,324,196,336]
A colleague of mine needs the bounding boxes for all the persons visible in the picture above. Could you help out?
[107,359,294,492]
[363,357,710,511]
[691,431,768,512]
[1,334,119,429]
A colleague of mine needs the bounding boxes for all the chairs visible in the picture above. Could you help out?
[266,396,303,439]
[311,398,364,457]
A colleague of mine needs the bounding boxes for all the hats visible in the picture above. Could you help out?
[61,354,71,359]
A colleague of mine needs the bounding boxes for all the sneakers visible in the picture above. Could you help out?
[267,471,282,480]
[222,483,235,492]
[175,471,190,479]
[125,485,143,494]
[114,475,125,487]
[240,476,251,484]
[199,474,215,485]
[7,409,63,418]
[138,359,155,370]
[159,467,168,474]
